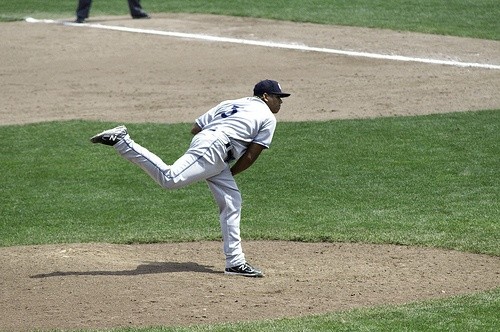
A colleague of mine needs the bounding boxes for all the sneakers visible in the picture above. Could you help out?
[89,124,128,146]
[225,262,265,277]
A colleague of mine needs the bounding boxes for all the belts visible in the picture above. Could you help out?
[210,129,233,162]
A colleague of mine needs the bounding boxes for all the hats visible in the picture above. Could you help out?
[254,79,291,98]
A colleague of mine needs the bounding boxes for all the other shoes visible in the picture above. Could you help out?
[76,16,85,23]
[134,13,149,19]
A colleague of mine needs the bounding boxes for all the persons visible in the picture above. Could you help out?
[89,78,283,279]
[73,0,150,22]
